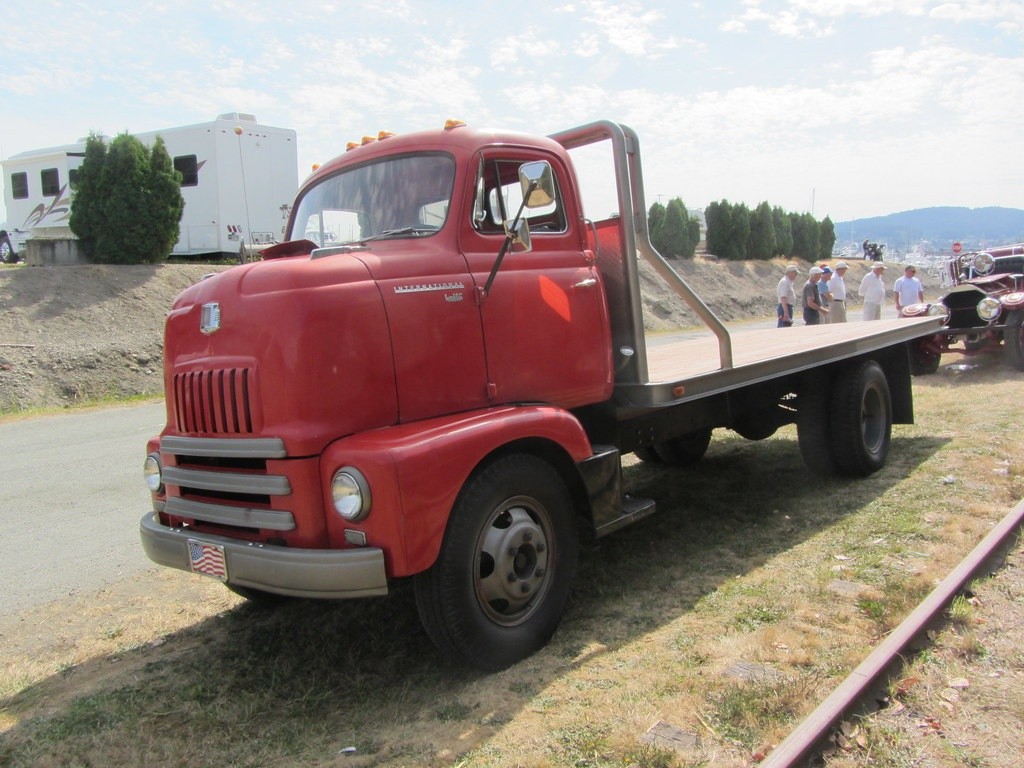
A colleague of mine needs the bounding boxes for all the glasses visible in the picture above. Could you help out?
[906,270,916,272]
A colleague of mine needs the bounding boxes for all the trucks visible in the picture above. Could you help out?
[2,112,297,265]
[138,120,951,675]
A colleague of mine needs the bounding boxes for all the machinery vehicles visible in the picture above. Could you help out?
[897,243,1024,376]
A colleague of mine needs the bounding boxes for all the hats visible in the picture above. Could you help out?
[809,267,824,274]
[785,265,801,273]
[871,262,887,269]
[836,262,849,269]
[822,266,834,274]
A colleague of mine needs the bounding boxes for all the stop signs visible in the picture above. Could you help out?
[952,243,961,253]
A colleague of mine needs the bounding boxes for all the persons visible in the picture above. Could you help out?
[858,261,887,322]
[818,263,829,283]
[829,261,848,323]
[893,263,924,318]
[777,265,800,327]
[861,239,870,261]
[817,266,833,325]
[800,266,830,326]
[869,242,877,260]
[876,245,885,261]
